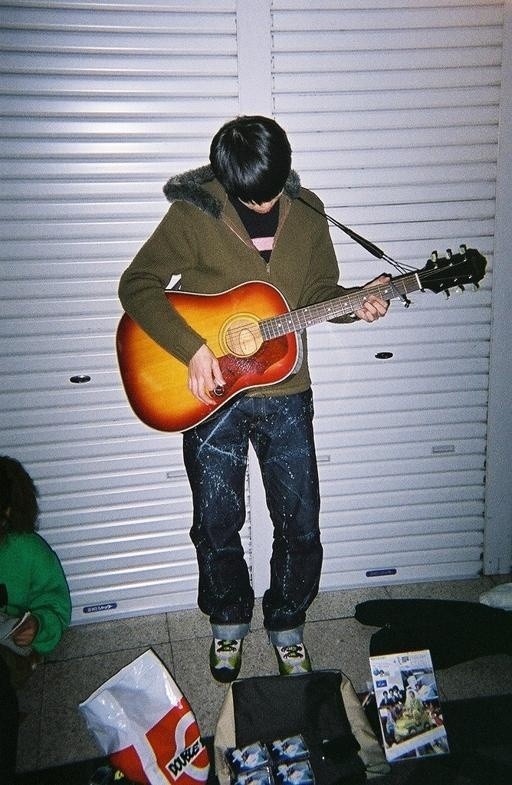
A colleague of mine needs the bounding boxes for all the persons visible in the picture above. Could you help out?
[1,455,75,784]
[377,669,443,749]
[117,113,394,678]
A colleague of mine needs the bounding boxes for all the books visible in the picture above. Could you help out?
[367,647,450,765]
[0,610,34,656]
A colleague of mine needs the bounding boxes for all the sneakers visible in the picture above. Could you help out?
[274,641,311,676]
[209,638,243,683]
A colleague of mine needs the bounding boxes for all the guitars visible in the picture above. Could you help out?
[116,244,486,435]
